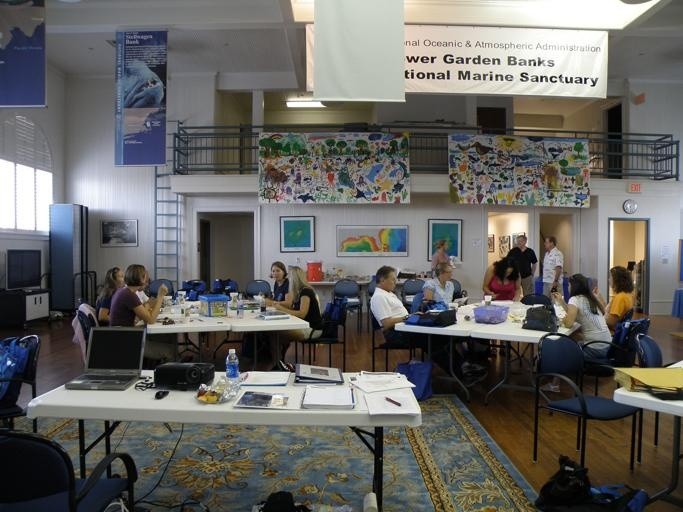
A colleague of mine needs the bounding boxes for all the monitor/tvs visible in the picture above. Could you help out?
[5,248,42,293]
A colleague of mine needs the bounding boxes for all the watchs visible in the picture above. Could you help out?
[405,315,409,320]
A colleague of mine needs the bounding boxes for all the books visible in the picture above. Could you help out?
[301,385,356,411]
[240,370,291,386]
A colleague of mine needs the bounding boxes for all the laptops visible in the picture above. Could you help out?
[65,326,149,391]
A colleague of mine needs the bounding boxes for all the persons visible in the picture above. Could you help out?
[431,240,452,277]
[96,266,125,328]
[481,258,522,357]
[258,261,291,308]
[110,264,175,365]
[542,235,564,300]
[370,267,489,387]
[591,265,634,337]
[506,235,538,297]
[539,272,612,392]
[422,263,454,310]
[123,269,156,311]
[259,265,323,370]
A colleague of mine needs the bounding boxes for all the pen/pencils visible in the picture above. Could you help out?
[241,373,249,381]
[385,396,401,406]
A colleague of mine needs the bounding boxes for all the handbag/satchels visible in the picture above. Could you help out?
[314,321,337,338]
[1,342,32,408]
[396,360,432,401]
[419,301,447,312]
[452,290,461,301]
[405,310,456,327]
[534,454,592,505]
[522,307,557,332]
[535,483,647,512]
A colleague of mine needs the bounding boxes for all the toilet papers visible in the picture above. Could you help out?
[363,492,378,511]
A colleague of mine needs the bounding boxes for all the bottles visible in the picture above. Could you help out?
[225,348,239,397]
[237,294,244,319]
[230,292,239,310]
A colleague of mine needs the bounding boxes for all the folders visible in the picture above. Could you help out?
[294,363,344,385]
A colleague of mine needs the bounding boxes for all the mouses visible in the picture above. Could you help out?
[155,389,169,400]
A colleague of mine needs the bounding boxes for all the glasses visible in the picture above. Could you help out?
[443,270,452,274]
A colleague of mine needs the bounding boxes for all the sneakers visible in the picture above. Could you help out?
[462,363,487,377]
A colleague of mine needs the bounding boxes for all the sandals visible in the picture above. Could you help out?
[539,382,561,393]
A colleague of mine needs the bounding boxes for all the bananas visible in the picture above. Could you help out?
[196,387,220,403]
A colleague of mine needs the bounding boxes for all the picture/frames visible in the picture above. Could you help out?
[488,233,525,258]
[426,218,464,264]
[281,215,317,253]
[335,225,410,258]
[99,218,139,248]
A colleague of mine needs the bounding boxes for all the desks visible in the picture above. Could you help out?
[26,272,683,512]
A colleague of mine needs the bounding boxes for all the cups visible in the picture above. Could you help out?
[484,296,491,305]
[163,296,172,308]
[261,298,267,312]
[178,291,186,304]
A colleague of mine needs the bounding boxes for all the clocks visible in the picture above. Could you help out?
[623,199,638,214]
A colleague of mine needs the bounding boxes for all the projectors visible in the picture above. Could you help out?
[153,360,215,391]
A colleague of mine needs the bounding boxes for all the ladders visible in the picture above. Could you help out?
[155,166,178,292]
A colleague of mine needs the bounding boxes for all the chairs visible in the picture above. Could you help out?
[0,431,137,512]
[1,334,40,433]
